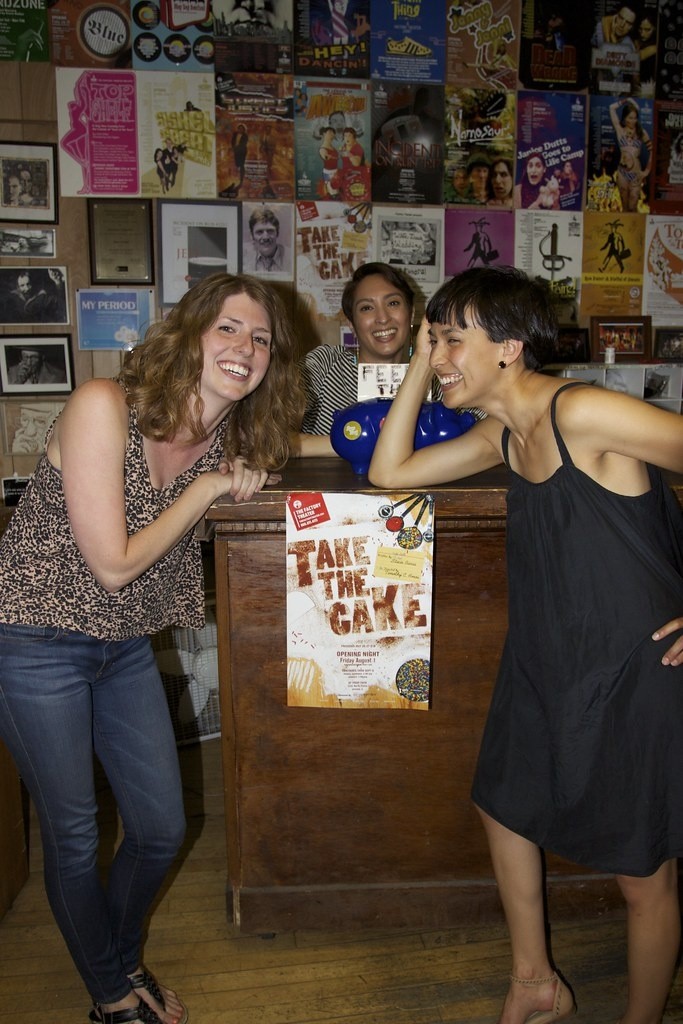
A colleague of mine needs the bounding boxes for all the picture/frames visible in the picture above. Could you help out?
[589,315,653,363]
[0,333,76,396]
[0,139,60,226]
[652,328,683,362]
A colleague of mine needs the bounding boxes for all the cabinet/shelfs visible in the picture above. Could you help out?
[205,456,629,933]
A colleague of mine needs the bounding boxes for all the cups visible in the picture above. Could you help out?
[604,347,615,365]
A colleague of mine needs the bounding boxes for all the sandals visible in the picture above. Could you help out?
[499,973,577,1024]
[90,964,188,1024]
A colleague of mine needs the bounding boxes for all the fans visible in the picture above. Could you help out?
[148,587,223,745]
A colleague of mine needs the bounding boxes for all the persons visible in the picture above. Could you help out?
[455,153,513,207]
[229,123,248,184]
[590,4,657,85]
[610,98,652,213]
[185,101,200,112]
[243,207,290,272]
[513,152,578,211]
[9,269,65,321]
[288,261,488,459]
[5,167,32,205]
[8,349,68,384]
[0,277,307,1024]
[369,267,683,1024]
[153,138,188,195]
[259,125,276,169]
